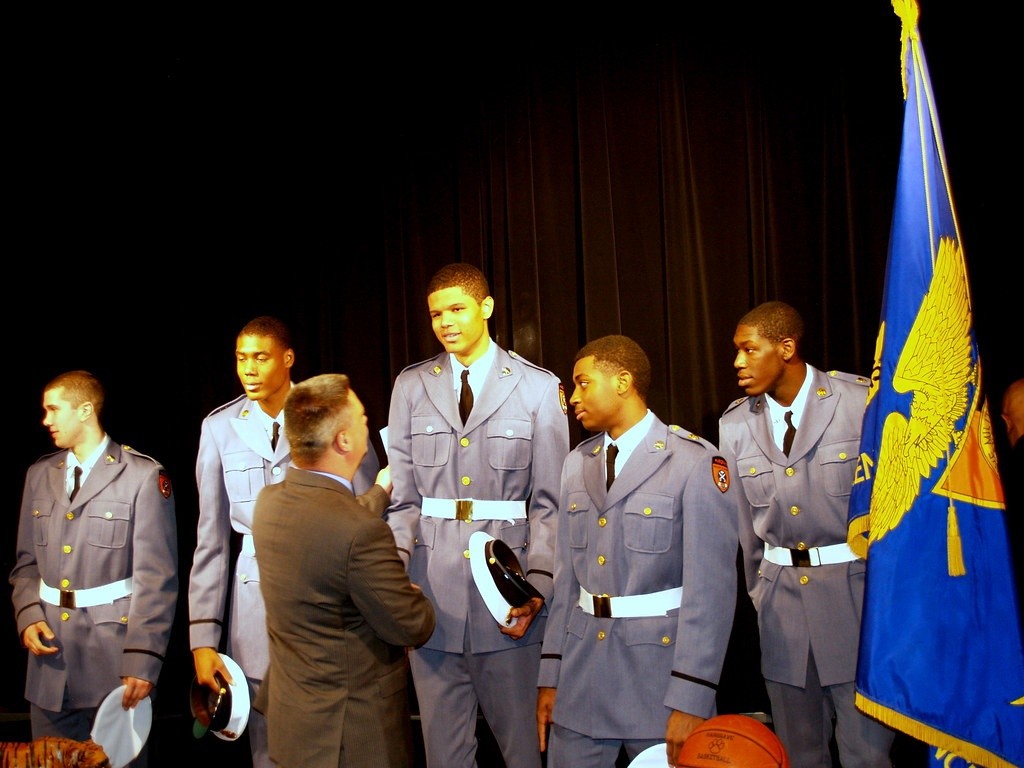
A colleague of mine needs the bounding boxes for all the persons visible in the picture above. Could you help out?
[998,380,1024,646]
[548,336,736,768]
[187,315,379,768]
[253,375,434,768]
[9,369,176,742]
[386,263,569,768]
[718,302,896,768]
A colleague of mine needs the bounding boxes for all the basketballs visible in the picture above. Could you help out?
[675,714,790,767]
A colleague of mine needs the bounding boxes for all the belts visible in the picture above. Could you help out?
[242,533,255,552]
[420,496,527,520]
[38,577,133,609]
[578,585,683,619]
[763,541,859,568]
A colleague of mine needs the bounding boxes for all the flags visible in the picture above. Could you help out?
[848,0,1024,768]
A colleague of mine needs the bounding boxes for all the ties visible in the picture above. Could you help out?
[782,410,798,458]
[270,422,282,452]
[69,465,83,504]
[606,443,619,493]
[459,370,474,428]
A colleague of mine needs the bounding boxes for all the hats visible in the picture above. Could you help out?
[628,742,677,768]
[90,684,152,768]
[189,651,251,741]
[469,531,545,629]
